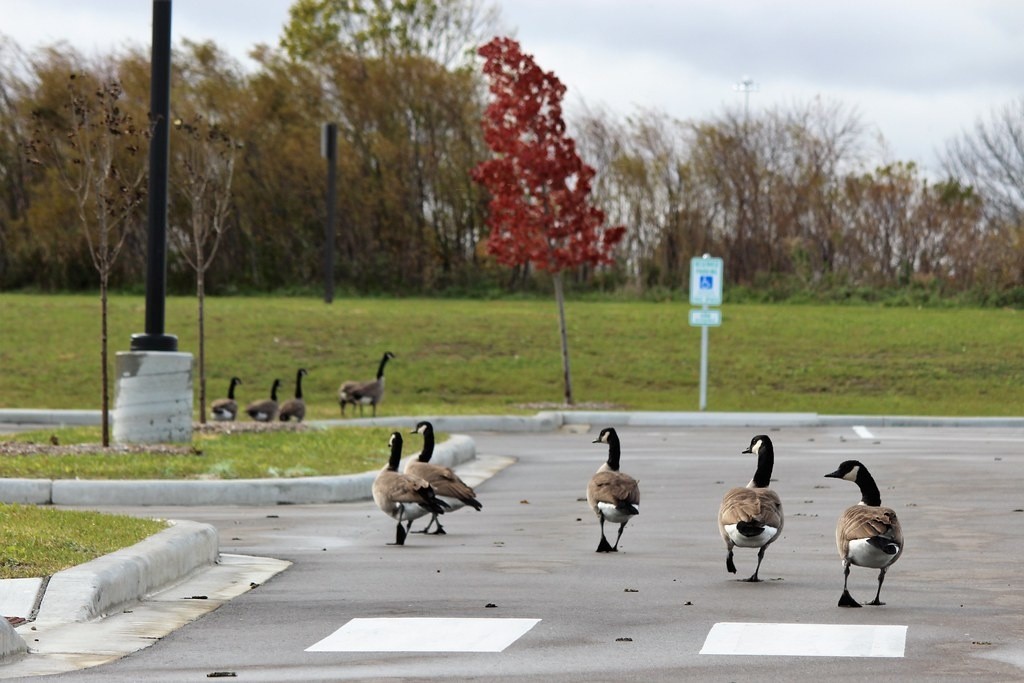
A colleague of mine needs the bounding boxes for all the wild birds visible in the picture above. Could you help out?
[243,377,281,421]
[409,420,485,536]
[371,431,448,541]
[278,368,309,422]
[339,351,396,417]
[823,459,905,609]
[212,374,243,420]
[585,426,642,553]
[717,433,785,583]
